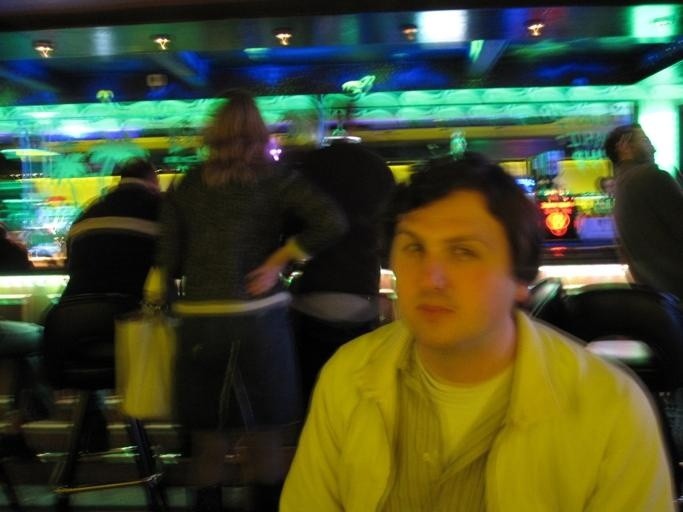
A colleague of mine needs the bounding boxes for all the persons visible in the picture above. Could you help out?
[274,150,678,510]
[39,158,164,386]
[600,123,682,320]
[270,93,399,377]
[140,92,347,437]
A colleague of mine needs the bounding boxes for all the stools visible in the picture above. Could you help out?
[0,276,681,511]
